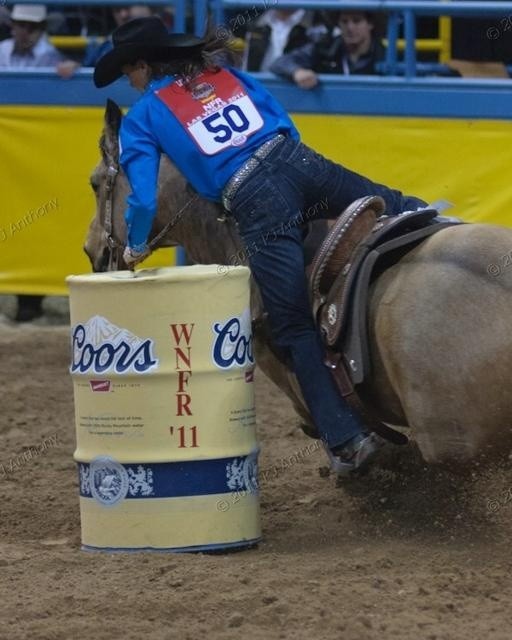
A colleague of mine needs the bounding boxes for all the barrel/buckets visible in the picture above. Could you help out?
[65,263,263,551]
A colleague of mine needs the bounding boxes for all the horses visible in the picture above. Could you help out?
[83,97,512,504]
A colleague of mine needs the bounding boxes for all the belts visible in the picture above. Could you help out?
[222,132,287,211]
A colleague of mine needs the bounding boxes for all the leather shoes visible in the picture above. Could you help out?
[332,434,385,486]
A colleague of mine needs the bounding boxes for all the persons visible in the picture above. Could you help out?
[92,16,430,481]
[0,2,388,92]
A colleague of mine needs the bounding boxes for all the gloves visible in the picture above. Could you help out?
[121,243,153,265]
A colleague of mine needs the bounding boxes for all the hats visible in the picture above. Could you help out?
[12,3,48,24]
[93,16,206,89]
